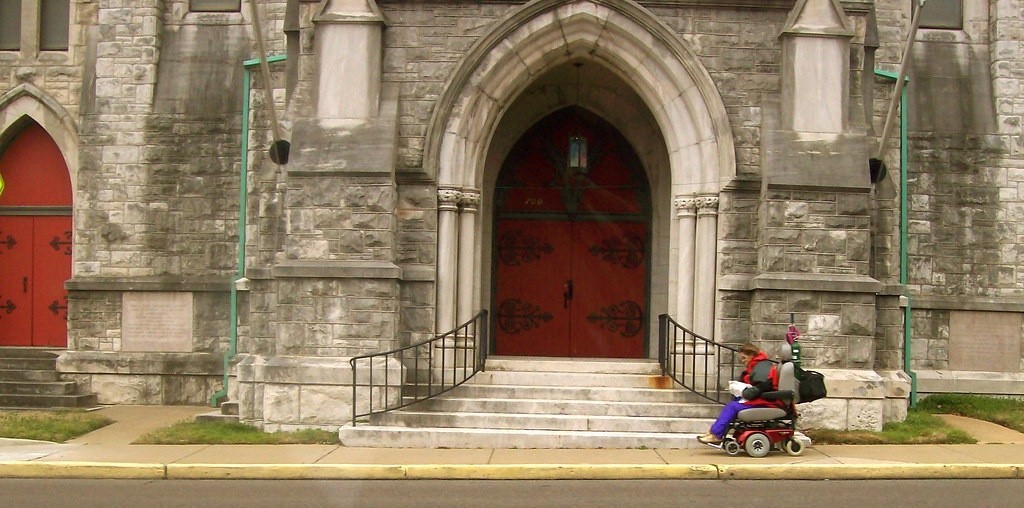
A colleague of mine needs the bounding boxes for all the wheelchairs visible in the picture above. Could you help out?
[708,313,812,458]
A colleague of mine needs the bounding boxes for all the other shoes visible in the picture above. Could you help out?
[697,432,721,445]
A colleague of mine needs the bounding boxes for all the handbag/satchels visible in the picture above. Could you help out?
[798,369,827,403]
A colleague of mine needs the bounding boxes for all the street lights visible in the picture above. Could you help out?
[873,69,918,408]
[210,53,288,408]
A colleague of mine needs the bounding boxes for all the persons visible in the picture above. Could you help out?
[698,343,779,445]
